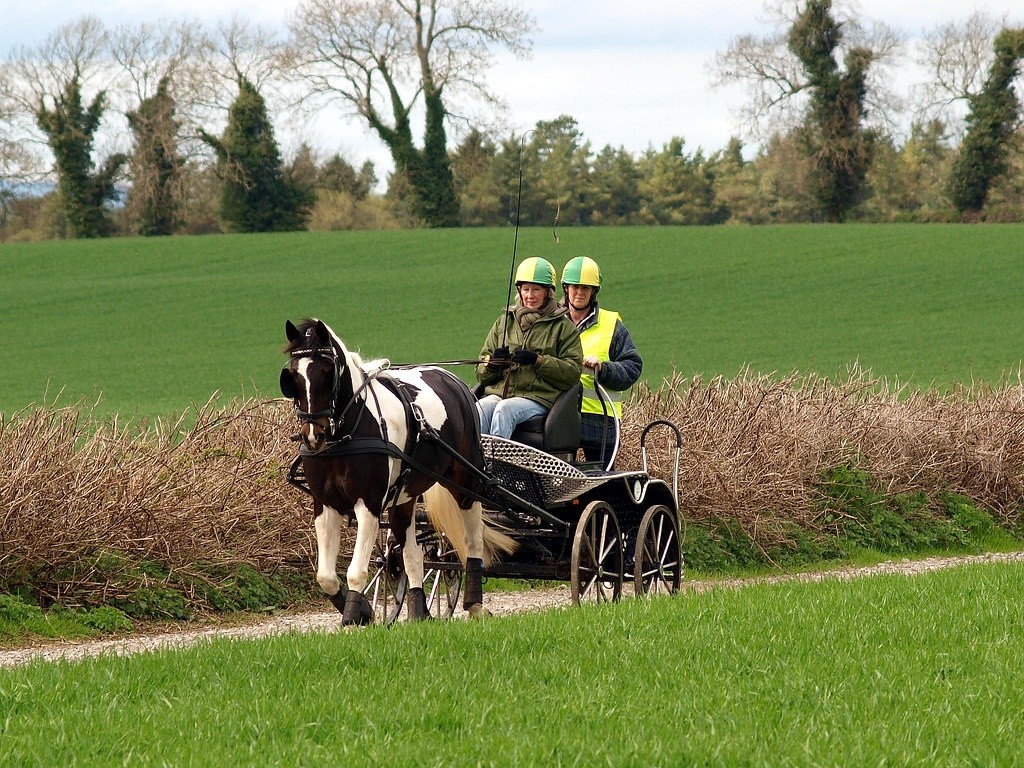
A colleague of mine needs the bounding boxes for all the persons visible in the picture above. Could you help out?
[475,256,584,440]
[557,256,642,471]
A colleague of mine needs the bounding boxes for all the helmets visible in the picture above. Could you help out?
[514,256,557,292]
[561,255,603,294]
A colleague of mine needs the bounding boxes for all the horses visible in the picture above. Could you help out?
[278,316,520,635]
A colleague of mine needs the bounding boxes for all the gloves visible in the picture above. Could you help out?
[493,345,510,360]
[512,350,538,365]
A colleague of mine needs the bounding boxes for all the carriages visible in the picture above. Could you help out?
[279,317,685,628]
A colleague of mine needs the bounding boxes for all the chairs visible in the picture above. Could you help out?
[471,379,584,465]
